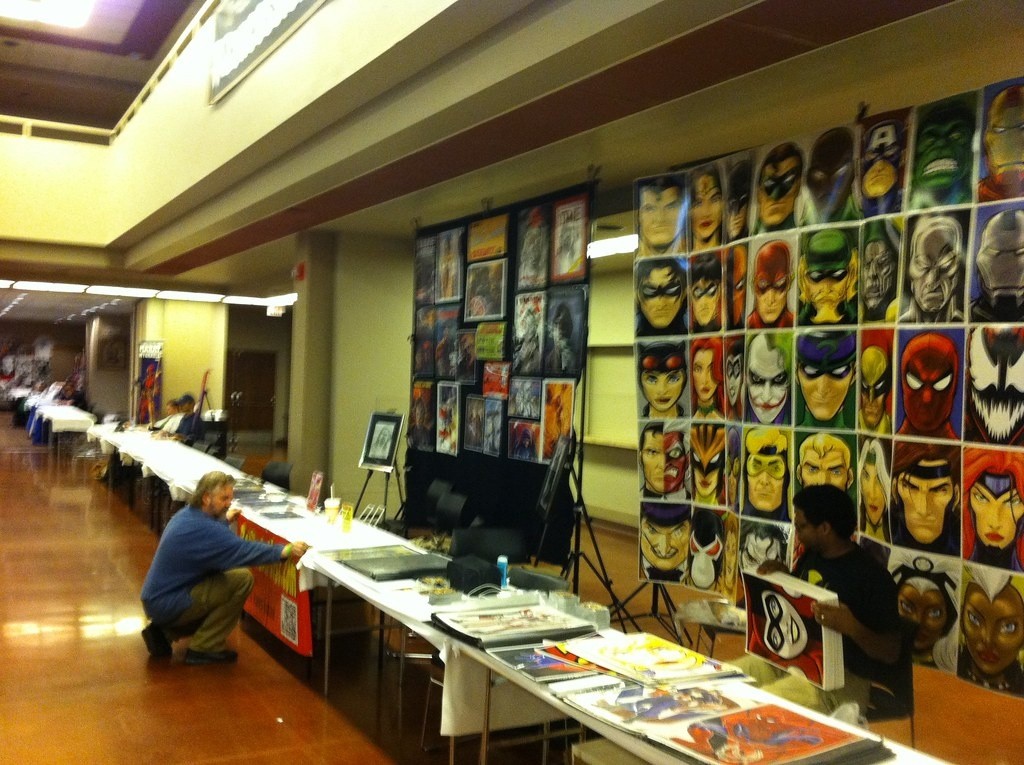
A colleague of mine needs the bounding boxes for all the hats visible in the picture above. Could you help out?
[176,395,194,405]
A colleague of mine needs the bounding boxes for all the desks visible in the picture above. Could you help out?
[88,421,949,765]
[11,387,94,431]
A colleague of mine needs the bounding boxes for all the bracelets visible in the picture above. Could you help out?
[285,545,292,555]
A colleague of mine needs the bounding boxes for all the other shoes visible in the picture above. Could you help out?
[185,648,238,664]
[141,626,172,658]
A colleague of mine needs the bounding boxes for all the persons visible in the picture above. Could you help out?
[728,483,900,726]
[141,471,308,661]
[53,382,88,449]
[146,394,203,505]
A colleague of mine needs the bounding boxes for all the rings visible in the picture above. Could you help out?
[822,614,824,620]
